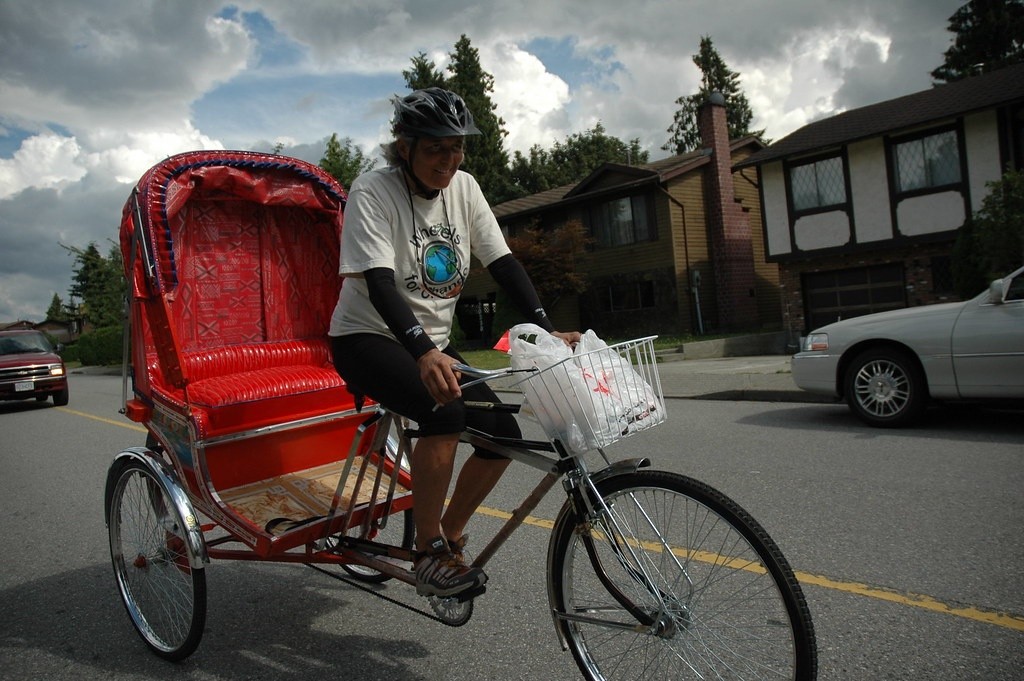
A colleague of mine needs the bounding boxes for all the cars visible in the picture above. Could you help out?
[790,261,1024,429]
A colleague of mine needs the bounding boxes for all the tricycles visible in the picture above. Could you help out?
[103,149,822,681]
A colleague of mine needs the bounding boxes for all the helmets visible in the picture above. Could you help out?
[394,85,483,139]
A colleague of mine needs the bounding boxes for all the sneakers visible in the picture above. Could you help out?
[414,533,490,598]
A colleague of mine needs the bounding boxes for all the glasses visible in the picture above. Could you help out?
[418,250,465,300]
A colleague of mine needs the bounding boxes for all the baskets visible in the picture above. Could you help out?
[508,335,669,461]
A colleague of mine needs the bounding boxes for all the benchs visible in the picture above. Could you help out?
[146,337,379,440]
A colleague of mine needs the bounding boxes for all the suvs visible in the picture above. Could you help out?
[0,330,71,407]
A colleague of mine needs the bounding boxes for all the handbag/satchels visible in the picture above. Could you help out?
[508,323,665,454]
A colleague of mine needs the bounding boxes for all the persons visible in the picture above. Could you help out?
[327,87,581,598]
